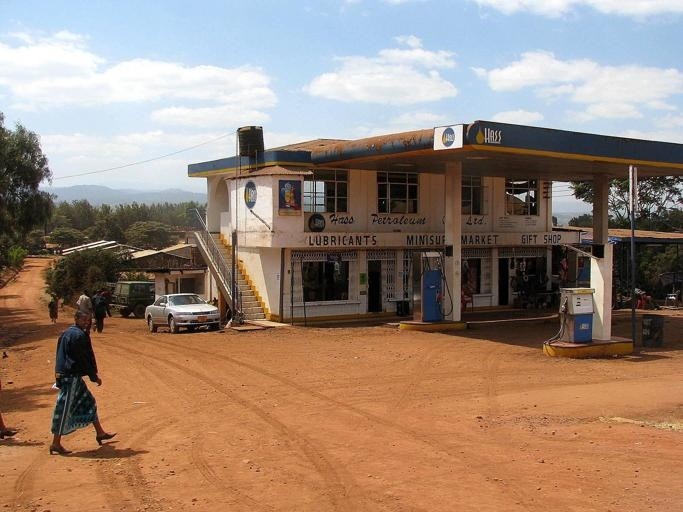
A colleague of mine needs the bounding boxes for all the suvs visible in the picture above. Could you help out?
[108,280,154,319]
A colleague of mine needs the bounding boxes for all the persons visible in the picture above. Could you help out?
[90,289,112,334]
[76,288,92,333]
[47,292,58,323]
[0,402,18,439]
[49,308,117,456]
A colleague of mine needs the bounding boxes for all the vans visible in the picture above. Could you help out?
[666,293,676,300]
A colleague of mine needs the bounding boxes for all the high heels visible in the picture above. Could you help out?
[0,430,17,439]
[50,445,72,454]
[96,432,117,445]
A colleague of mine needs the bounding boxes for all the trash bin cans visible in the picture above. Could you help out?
[642,314,664,348]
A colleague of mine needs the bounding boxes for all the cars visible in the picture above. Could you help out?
[142,292,220,333]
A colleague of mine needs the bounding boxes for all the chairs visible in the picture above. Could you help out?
[461,282,474,311]
